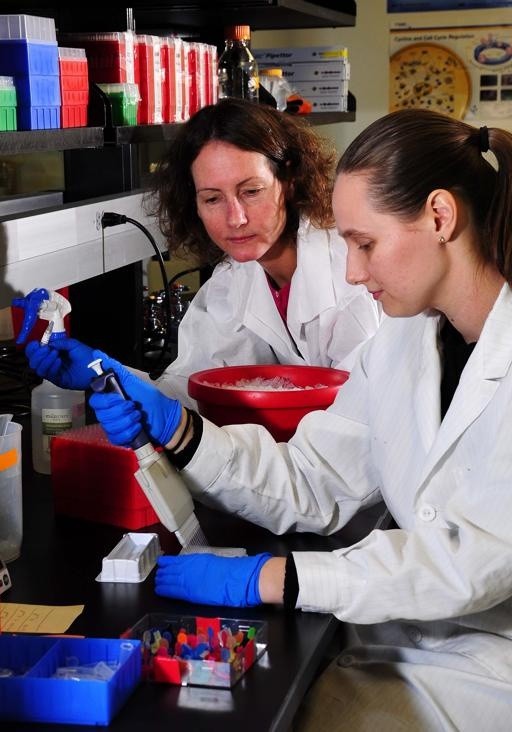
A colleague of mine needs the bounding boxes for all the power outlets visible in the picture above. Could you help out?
[93,206,120,231]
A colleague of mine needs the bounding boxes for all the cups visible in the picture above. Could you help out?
[1,418,27,563]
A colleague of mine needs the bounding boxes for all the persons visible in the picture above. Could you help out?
[24,93,391,444]
[87,107,511,732]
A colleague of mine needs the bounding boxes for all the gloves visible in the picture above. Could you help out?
[24,339,100,391]
[153,551,273,608]
[88,349,182,450]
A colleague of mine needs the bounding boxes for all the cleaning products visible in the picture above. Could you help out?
[12,288,87,475]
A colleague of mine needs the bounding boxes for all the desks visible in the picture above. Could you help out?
[0,498,393,730]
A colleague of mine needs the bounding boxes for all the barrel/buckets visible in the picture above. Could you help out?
[0,415,23,564]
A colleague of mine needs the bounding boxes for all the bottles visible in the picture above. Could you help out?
[219,24,259,103]
[257,69,290,113]
